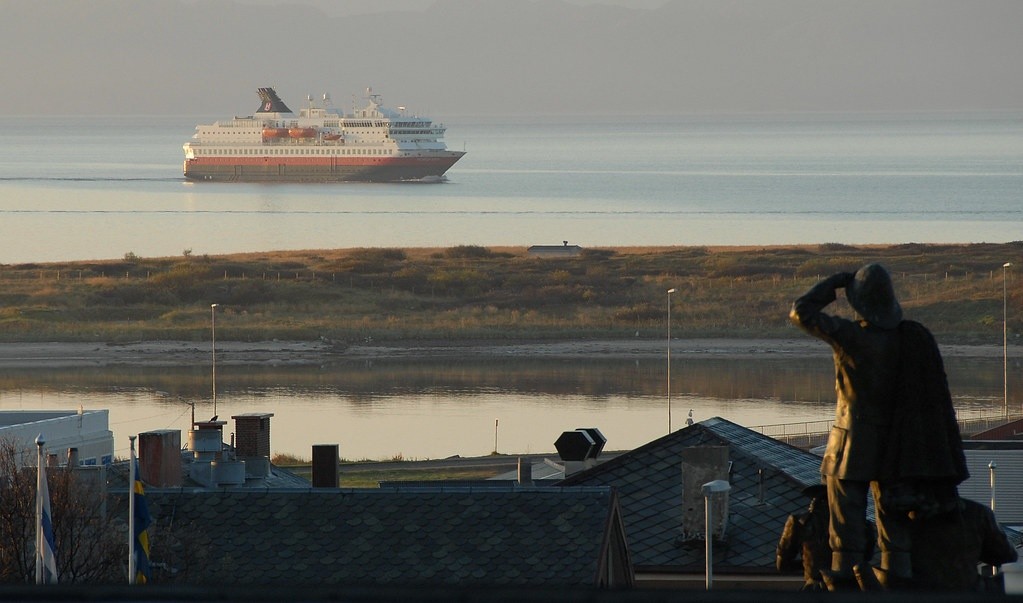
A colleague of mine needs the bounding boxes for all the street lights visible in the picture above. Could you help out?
[212,303,220,420]
[668,288,678,435]
[1003,262,1013,419]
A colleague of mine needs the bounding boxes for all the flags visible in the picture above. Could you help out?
[134,457,153,586]
[40,467,59,583]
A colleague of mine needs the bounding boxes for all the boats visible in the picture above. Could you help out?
[183,86,466,182]
[287,129,315,138]
[262,128,288,137]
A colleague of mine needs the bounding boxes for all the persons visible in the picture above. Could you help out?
[773,262,1019,603]
